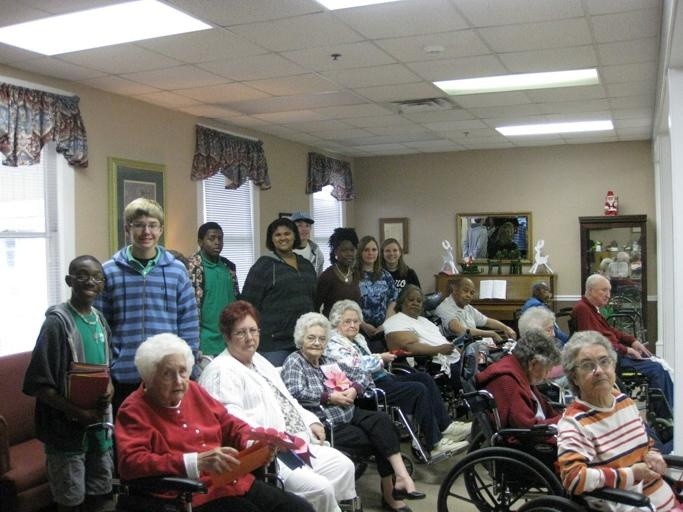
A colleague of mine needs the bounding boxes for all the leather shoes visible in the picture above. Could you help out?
[392,487,426,500]
[411,443,424,460]
[381,496,413,512]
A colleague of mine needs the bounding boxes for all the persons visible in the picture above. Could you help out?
[604,191,618,216]
[521,281,570,348]
[466,328,562,485]
[287,212,326,278]
[186,220,242,366]
[554,329,682,510]
[379,238,420,289]
[112,332,316,511]
[516,306,575,408]
[353,234,399,354]
[570,274,673,410]
[280,311,427,512]
[234,216,320,369]
[20,254,115,510]
[313,226,362,316]
[433,277,518,355]
[323,298,473,463]
[613,251,641,294]
[382,286,465,399]
[599,258,616,284]
[459,216,529,262]
[92,196,202,473]
[191,298,359,512]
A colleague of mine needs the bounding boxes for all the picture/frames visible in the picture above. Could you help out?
[106,154,167,263]
[375,216,409,256]
[630,227,641,233]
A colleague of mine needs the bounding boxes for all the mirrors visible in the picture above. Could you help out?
[452,210,534,266]
[575,212,649,343]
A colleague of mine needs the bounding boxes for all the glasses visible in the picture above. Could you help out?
[231,328,261,339]
[70,275,105,284]
[129,222,162,229]
[573,357,614,372]
[342,320,362,326]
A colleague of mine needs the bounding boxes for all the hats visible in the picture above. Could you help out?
[291,211,315,224]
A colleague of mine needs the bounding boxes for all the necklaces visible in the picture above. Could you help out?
[65,300,99,325]
[386,266,398,269]
[335,263,351,283]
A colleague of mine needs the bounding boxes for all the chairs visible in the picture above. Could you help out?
[0,350,51,512]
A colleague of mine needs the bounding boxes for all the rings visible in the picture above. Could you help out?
[321,428,325,433]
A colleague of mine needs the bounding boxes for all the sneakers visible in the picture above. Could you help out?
[440,421,473,442]
[430,437,470,460]
